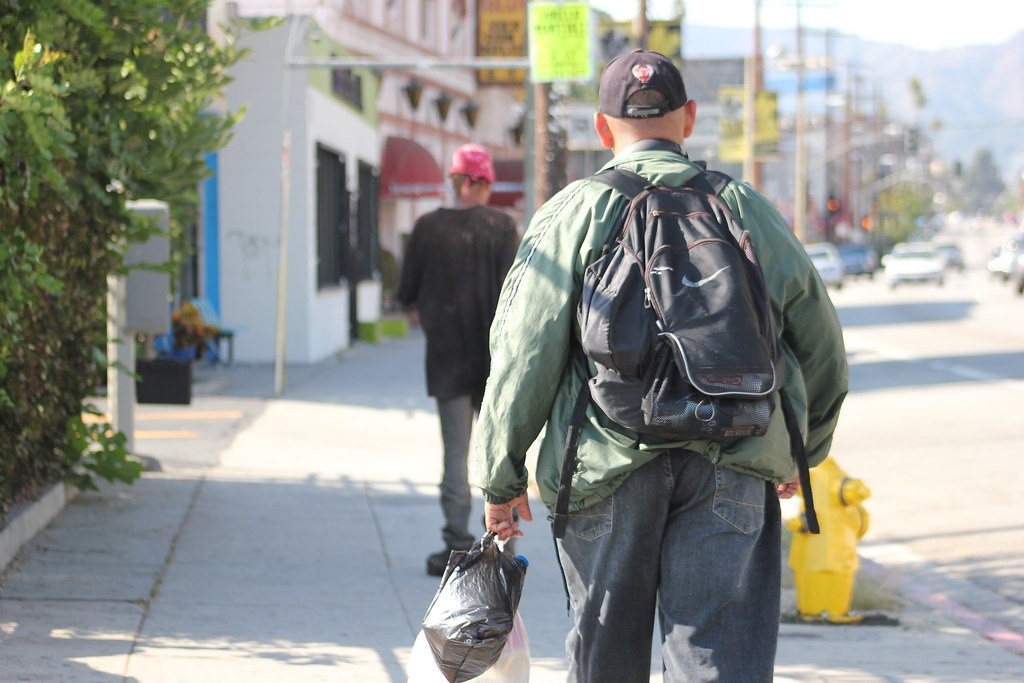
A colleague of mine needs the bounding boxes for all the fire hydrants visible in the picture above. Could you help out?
[783,459,869,620]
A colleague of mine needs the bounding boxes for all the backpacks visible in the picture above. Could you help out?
[551,169,823,538]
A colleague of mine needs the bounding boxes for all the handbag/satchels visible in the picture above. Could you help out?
[406,530,532,683]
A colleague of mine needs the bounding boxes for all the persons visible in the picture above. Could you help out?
[469,48,849,683]
[397,144,520,578]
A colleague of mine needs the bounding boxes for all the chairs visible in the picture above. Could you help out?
[190,296,249,367]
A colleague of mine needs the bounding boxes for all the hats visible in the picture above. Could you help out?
[451,144,494,185]
[599,48,688,118]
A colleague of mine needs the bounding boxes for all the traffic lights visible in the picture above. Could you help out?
[823,191,843,216]
[858,215,874,232]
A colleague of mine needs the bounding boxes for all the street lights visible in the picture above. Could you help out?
[790,125,907,243]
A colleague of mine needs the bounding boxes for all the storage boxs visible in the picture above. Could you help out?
[135,357,193,405]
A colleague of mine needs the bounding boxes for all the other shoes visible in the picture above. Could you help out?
[426,547,455,576]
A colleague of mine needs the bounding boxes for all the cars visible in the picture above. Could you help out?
[881,242,947,292]
[802,241,876,289]
[935,235,1024,293]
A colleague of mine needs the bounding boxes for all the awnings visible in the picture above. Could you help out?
[487,160,524,207]
[378,136,448,201]
